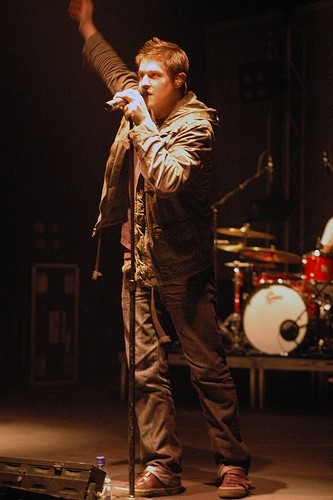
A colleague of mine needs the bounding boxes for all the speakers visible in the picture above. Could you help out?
[30,264,78,387]
[0,456,107,500]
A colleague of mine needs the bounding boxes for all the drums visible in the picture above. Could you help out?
[302,252,333,289]
[251,270,299,285]
[240,281,321,358]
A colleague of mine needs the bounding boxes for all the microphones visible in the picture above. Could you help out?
[103,87,147,112]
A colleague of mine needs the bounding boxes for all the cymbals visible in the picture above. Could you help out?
[215,242,304,264]
[217,227,277,241]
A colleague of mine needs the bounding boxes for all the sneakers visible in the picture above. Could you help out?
[217,469,252,498]
[111,470,182,497]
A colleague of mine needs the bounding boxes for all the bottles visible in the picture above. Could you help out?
[95,456,112,500]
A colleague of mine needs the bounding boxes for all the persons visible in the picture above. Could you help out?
[69,0,252,496]
